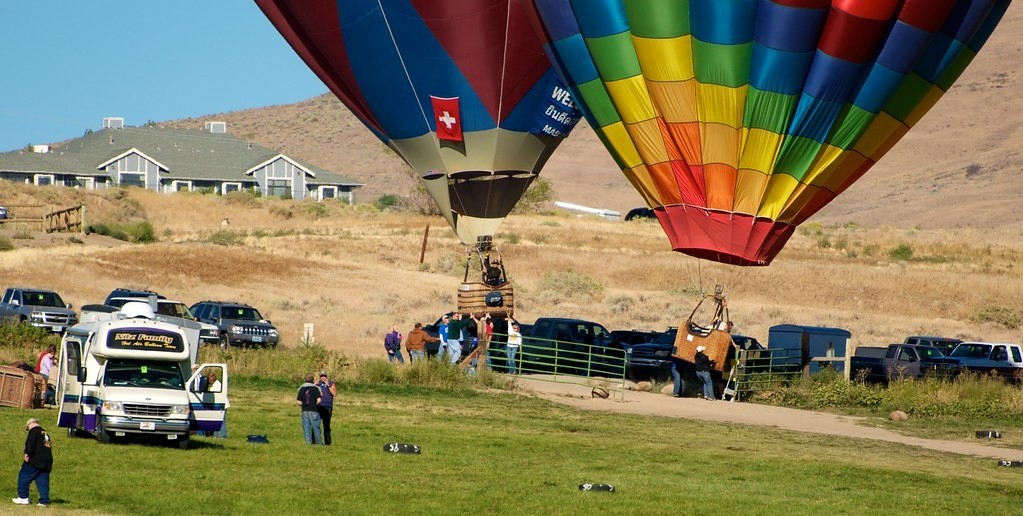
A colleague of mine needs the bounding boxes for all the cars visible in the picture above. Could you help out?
[624,207,657,222]
[723,335,771,375]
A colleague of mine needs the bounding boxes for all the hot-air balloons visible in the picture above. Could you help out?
[529,1,1014,375]
[251,2,583,320]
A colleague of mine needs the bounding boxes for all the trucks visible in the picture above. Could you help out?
[55,316,230,450]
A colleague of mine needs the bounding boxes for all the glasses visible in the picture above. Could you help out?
[320,375,326,377]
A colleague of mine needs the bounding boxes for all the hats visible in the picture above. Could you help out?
[191,364,199,369]
[442,316,449,322]
[25,418,39,430]
[318,370,327,376]
[491,259,500,264]
[512,322,520,325]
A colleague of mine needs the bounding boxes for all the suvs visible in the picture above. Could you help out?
[104,288,178,316]
[188,300,278,351]
[611,329,664,347]
[625,326,705,383]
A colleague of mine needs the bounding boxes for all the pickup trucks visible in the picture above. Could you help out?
[848,342,965,389]
[79,297,221,346]
[0,288,78,337]
[420,310,462,356]
[853,335,964,359]
[495,319,629,377]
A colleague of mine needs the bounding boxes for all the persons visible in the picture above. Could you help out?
[671,355,687,398]
[297,374,324,446]
[35,343,58,408]
[383,310,523,375]
[207,372,230,440]
[693,346,717,401]
[11,418,53,508]
[187,364,206,436]
[315,370,337,446]
[706,320,737,348]
[483,251,502,287]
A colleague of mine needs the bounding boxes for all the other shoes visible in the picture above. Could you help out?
[36,503,44,507]
[11,498,29,505]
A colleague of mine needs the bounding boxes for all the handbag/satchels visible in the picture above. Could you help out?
[485,292,503,307]
[247,433,269,443]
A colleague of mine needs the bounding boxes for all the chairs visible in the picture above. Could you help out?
[579,329,586,338]
[23,294,27,305]
[30,294,39,305]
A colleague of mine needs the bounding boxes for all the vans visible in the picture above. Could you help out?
[945,343,1023,376]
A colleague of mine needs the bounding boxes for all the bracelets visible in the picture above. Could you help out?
[328,386,331,388]
